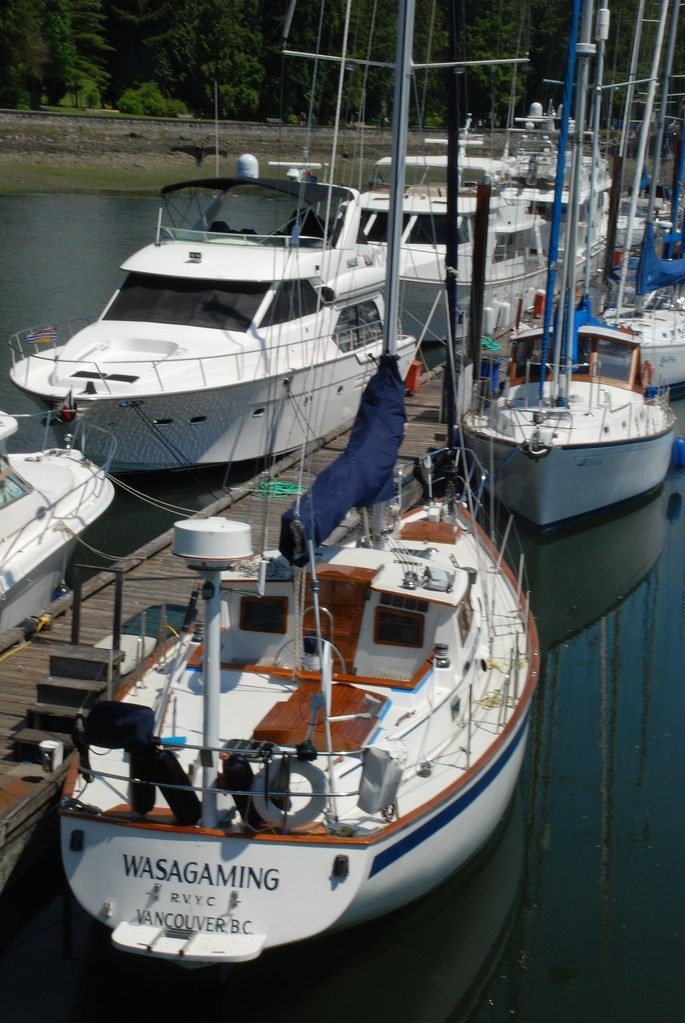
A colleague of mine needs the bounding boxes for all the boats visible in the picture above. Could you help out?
[249,776,535,1023]
[0,407,118,651]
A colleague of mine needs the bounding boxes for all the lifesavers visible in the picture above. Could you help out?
[250,756,330,832]
[640,359,653,388]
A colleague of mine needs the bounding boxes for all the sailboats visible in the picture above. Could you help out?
[494,481,670,974]
[0,0,683,972]
